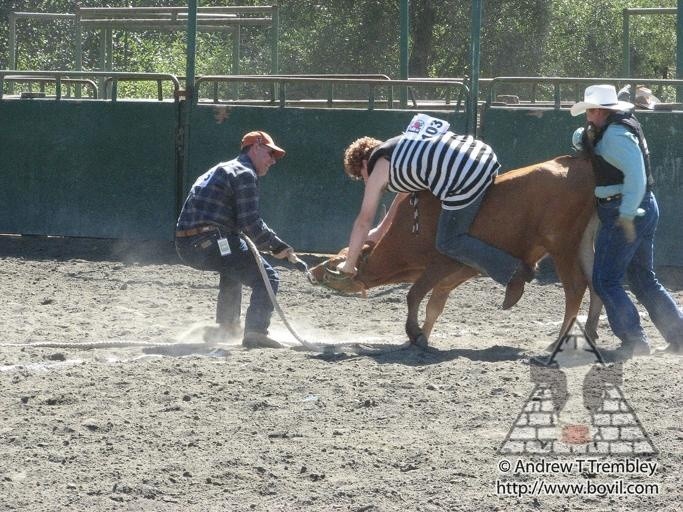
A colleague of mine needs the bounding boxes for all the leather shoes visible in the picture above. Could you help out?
[501,262,536,311]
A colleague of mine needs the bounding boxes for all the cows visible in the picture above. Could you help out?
[306,119,605,351]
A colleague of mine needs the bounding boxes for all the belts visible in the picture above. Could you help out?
[595,184,652,203]
[174,224,220,238]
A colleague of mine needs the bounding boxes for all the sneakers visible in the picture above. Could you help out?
[217,323,285,347]
[606,327,683,357]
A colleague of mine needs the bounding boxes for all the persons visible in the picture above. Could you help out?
[569,82,682,359]
[335,126,536,310]
[173,130,299,351]
[617,83,661,111]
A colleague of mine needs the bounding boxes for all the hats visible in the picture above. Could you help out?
[239,130,287,160]
[569,84,635,117]
